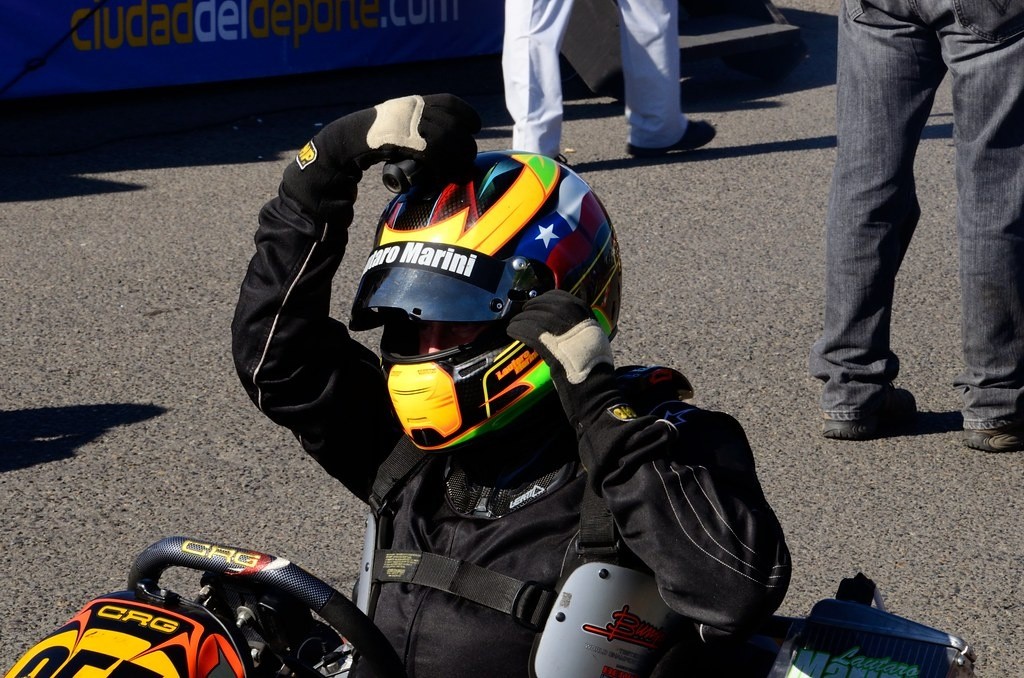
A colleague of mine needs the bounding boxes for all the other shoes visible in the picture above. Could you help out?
[627,120,716,157]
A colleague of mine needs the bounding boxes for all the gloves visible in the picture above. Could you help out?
[281,93,478,215]
[507,289,614,422]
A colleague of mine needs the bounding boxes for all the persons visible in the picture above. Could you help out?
[810,0,1024,452]
[501,0,718,162]
[231,91,792,678]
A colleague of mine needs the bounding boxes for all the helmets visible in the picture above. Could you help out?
[347,150,622,451]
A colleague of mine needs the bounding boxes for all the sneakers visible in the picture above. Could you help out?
[961,419,1024,453]
[823,387,917,440]
